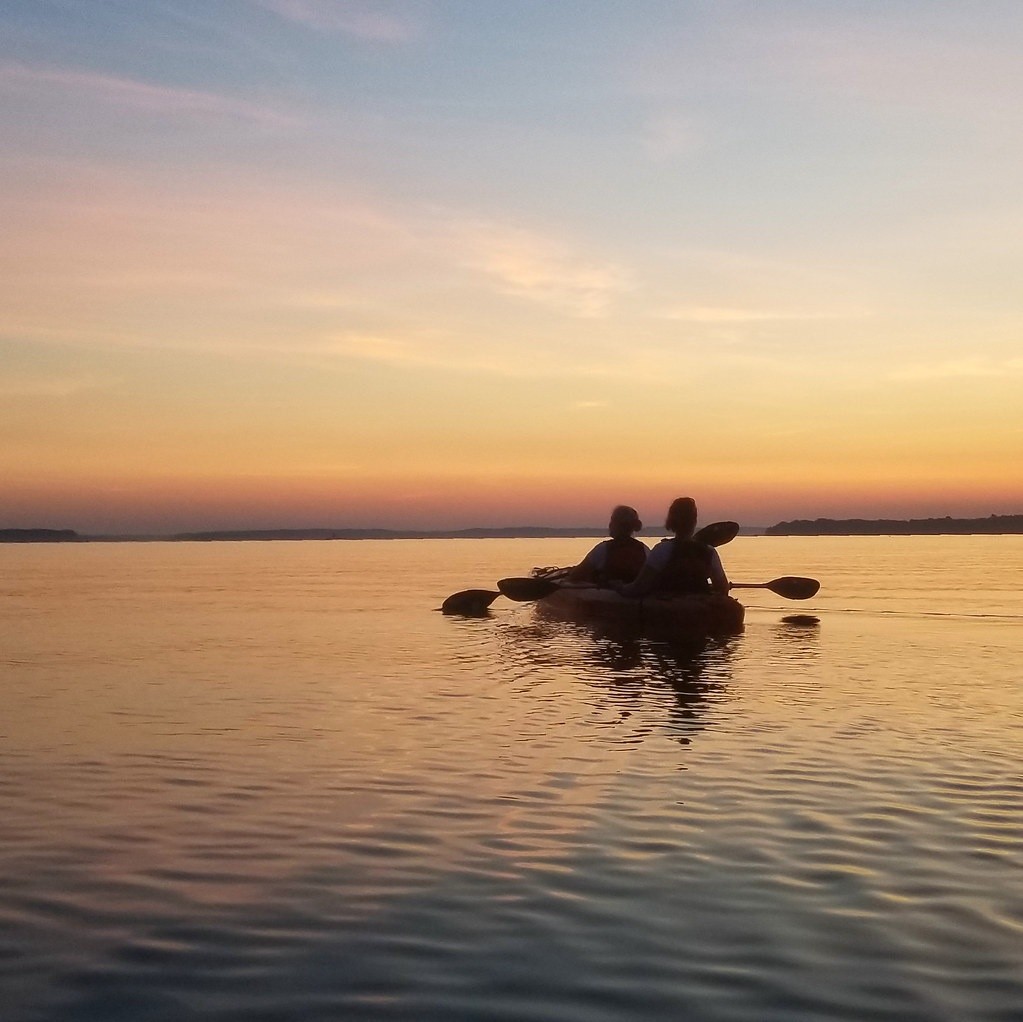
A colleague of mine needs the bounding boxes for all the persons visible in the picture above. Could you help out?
[563,506,650,584]
[629,497,729,597]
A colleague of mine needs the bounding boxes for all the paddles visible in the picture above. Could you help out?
[495,576,821,603]
[441,521,740,610]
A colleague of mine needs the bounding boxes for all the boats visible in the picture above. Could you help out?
[529,563,745,630]
